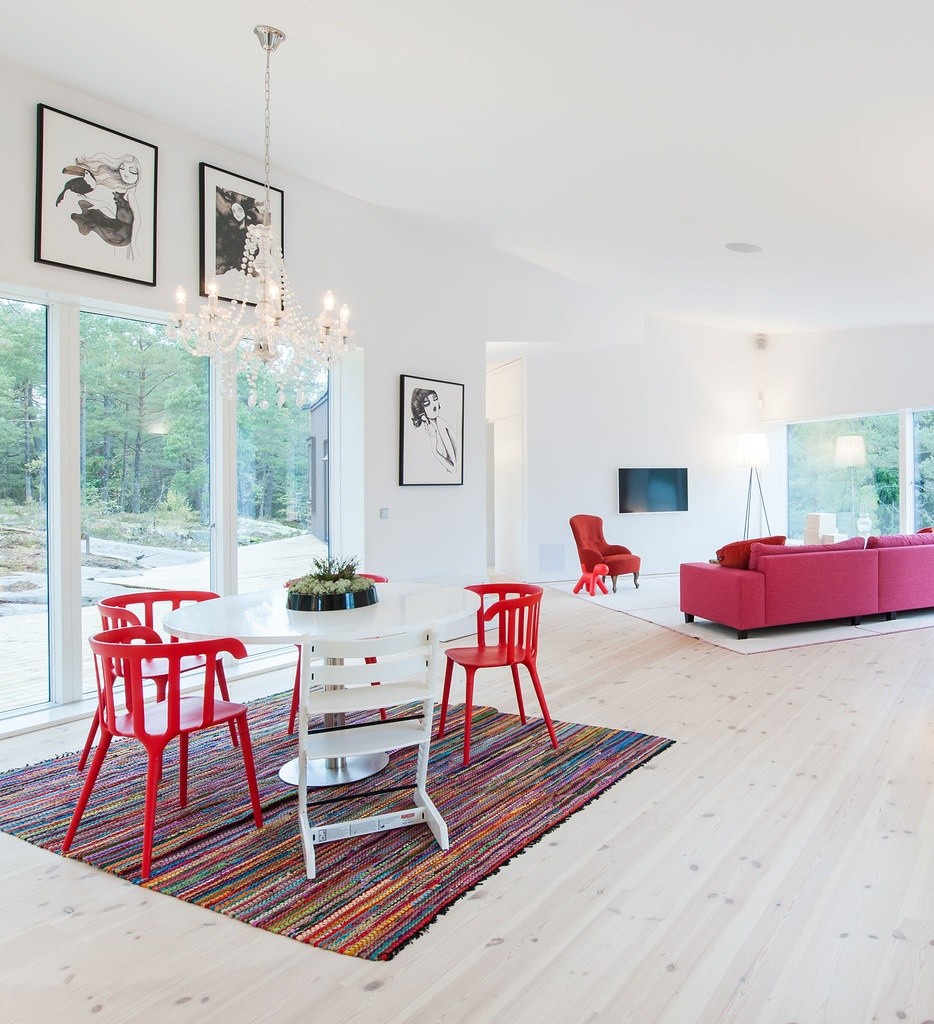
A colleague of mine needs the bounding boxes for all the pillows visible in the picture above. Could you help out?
[716,535,786,569]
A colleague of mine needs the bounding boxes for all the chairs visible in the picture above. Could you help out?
[569,514,641,593]
[298,627,449,879]
[439,583,558,767]
[62,624,263,879]
[76,590,238,778]
[285,574,389,734]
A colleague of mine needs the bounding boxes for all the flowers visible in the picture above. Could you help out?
[286,550,376,595]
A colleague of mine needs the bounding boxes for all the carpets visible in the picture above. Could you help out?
[549,574,934,655]
[0,684,677,962]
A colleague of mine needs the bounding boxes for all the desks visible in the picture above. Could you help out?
[163,582,481,787]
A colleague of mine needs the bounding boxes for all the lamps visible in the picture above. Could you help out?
[741,434,772,540]
[834,435,866,536]
[165,24,349,412]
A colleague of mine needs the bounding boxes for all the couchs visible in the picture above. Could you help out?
[679,532,934,640]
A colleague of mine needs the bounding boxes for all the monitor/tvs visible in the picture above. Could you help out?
[619,467,688,513]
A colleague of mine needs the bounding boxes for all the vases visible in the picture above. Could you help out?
[286,587,379,612]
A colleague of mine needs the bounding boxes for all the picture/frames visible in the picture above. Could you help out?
[34,103,158,288]
[399,374,465,486]
[199,161,284,312]
